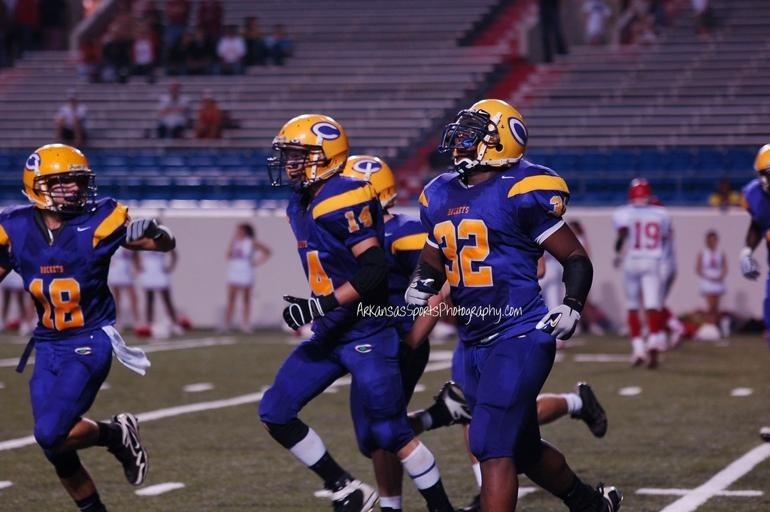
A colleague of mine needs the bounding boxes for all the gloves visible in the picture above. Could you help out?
[125,217,159,245]
[404,275,434,307]
[739,255,760,280]
[536,304,580,341]
[283,292,328,330]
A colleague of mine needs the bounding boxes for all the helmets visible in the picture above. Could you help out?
[339,155,398,211]
[273,112,347,185]
[24,144,90,213]
[454,98,528,178]
[754,143,770,171]
[630,178,651,205]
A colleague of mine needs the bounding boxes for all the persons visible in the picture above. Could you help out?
[568,220,617,333]
[687,0,714,40]
[1,269,25,327]
[158,82,192,135]
[695,230,727,327]
[537,0,569,64]
[217,24,246,75]
[240,16,266,65]
[340,155,473,512]
[258,114,454,512]
[27,295,38,324]
[14,0,38,50]
[106,248,140,334]
[738,145,770,442]
[272,24,293,65]
[461,254,608,512]
[613,177,671,369]
[709,178,739,206]
[404,99,623,512]
[195,98,221,139]
[614,0,657,47]
[221,224,272,336]
[133,251,185,341]
[584,2,609,45]
[54,83,88,149]
[68,0,215,83]
[648,196,688,352]
[0,143,176,512]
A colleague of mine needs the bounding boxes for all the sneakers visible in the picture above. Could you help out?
[107,412,148,486]
[135,326,153,337]
[760,426,770,441]
[177,318,195,330]
[316,480,380,511]
[647,346,660,370]
[571,382,608,437]
[631,355,646,366]
[598,486,622,510]
[435,379,472,425]
